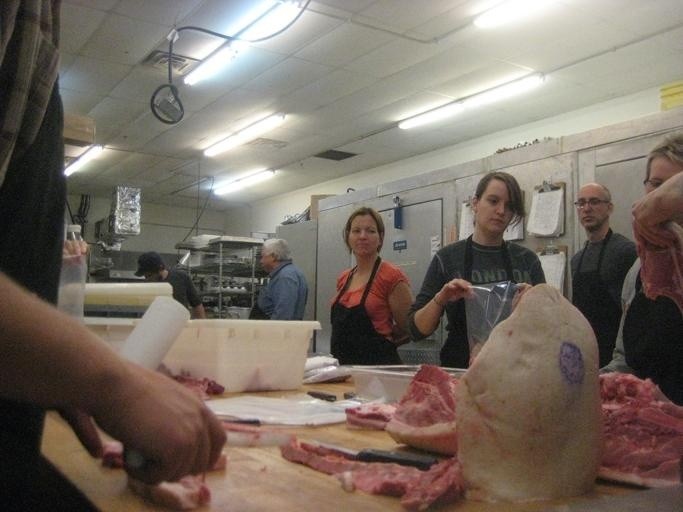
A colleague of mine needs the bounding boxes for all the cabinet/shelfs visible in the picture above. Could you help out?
[277,222,317,351]
[173,237,270,319]
[316,107,681,367]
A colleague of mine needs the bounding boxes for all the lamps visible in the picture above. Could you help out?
[471,1,556,29]
[395,72,545,130]
[204,112,286,158]
[182,1,295,86]
[64,145,103,176]
[215,167,275,196]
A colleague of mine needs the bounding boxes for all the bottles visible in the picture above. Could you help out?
[56,224,87,321]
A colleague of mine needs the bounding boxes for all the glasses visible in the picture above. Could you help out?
[575,199,610,208]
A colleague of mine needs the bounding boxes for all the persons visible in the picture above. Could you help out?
[128,247,208,320]
[623,128,682,408]
[0,0,227,510]
[248,237,308,320]
[406,172,546,368]
[563,182,637,368]
[329,207,413,364]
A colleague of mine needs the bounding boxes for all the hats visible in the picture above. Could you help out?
[134,252,161,276]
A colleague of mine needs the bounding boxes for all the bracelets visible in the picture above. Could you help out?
[433,294,447,310]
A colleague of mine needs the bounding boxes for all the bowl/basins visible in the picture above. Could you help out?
[226,306,250,319]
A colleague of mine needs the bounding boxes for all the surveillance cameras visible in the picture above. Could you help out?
[154,99,182,122]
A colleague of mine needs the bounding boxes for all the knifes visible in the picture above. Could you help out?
[306,436,438,471]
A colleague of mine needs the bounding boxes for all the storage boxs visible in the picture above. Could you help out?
[72,308,322,394]
